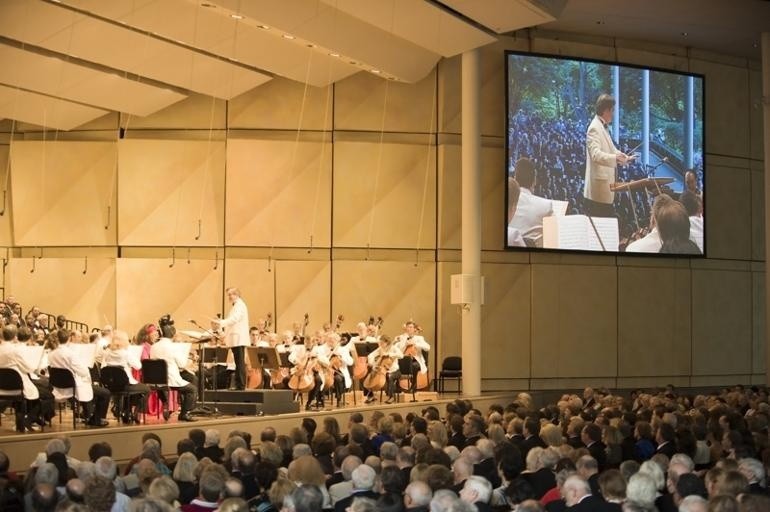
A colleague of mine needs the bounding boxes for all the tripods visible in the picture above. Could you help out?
[192,345,225,416]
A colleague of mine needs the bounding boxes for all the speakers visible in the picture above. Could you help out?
[450,274,473,304]
[481,276,485,305]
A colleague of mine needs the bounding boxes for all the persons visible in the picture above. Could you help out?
[207,286,252,391]
[508,94,705,257]
[202,314,433,411]
[0,294,201,431]
[0,380,769,512]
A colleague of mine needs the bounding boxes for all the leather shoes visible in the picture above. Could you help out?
[111,405,140,424]
[22,418,45,432]
[178,414,198,421]
[80,410,109,426]
[163,408,170,421]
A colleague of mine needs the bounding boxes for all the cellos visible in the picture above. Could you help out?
[215,314,432,393]
